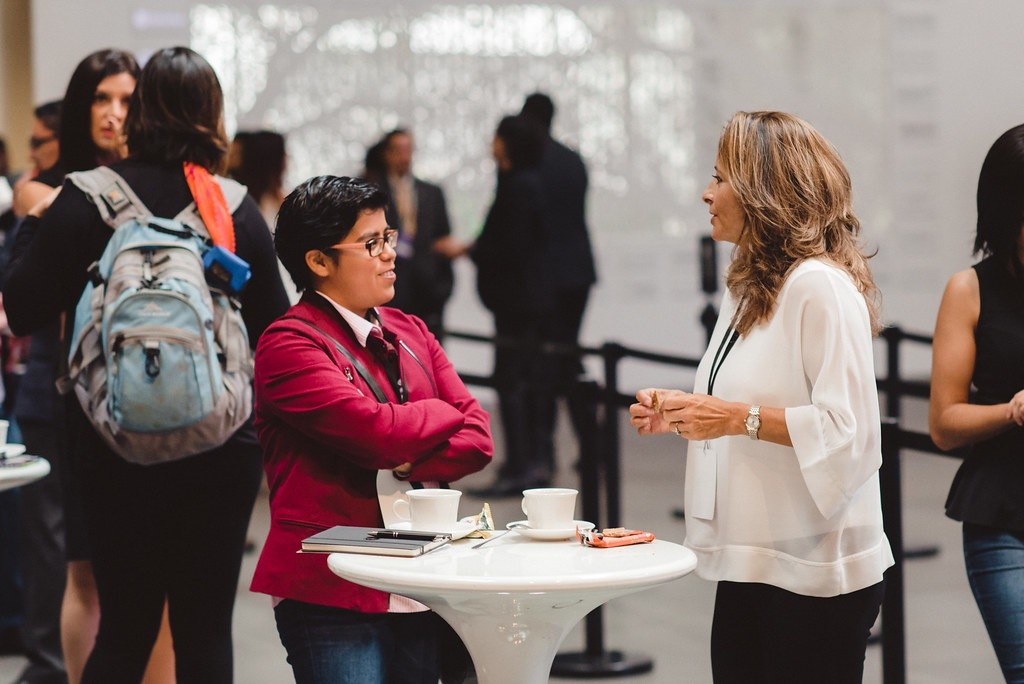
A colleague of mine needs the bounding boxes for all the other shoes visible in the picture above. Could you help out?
[468,472,544,499]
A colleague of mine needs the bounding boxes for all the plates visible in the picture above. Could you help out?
[0,443,27,460]
[506,520,596,540]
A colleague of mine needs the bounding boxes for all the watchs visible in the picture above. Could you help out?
[743,404,762,440]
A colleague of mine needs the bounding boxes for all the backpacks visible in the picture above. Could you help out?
[55,165,257,467]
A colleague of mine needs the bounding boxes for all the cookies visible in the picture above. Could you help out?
[602,527,627,537]
[651,391,662,414]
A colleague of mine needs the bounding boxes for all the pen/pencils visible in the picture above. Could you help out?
[369,531,452,540]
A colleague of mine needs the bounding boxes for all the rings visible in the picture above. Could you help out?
[675,422,680,435]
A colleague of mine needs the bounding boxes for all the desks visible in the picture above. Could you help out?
[327,530,700,684]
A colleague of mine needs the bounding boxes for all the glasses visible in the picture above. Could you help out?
[30,135,57,149]
[317,229,399,257]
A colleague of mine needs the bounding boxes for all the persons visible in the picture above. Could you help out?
[630,110,895,684]
[434,93,598,499]
[929,122,1024,684]
[0,45,291,684]
[350,128,456,346]
[249,177,495,683]
[225,128,288,553]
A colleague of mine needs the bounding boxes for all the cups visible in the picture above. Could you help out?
[0,420,9,449]
[522,488,580,530]
[406,488,462,534]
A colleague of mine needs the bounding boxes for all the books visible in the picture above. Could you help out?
[302,525,453,558]
